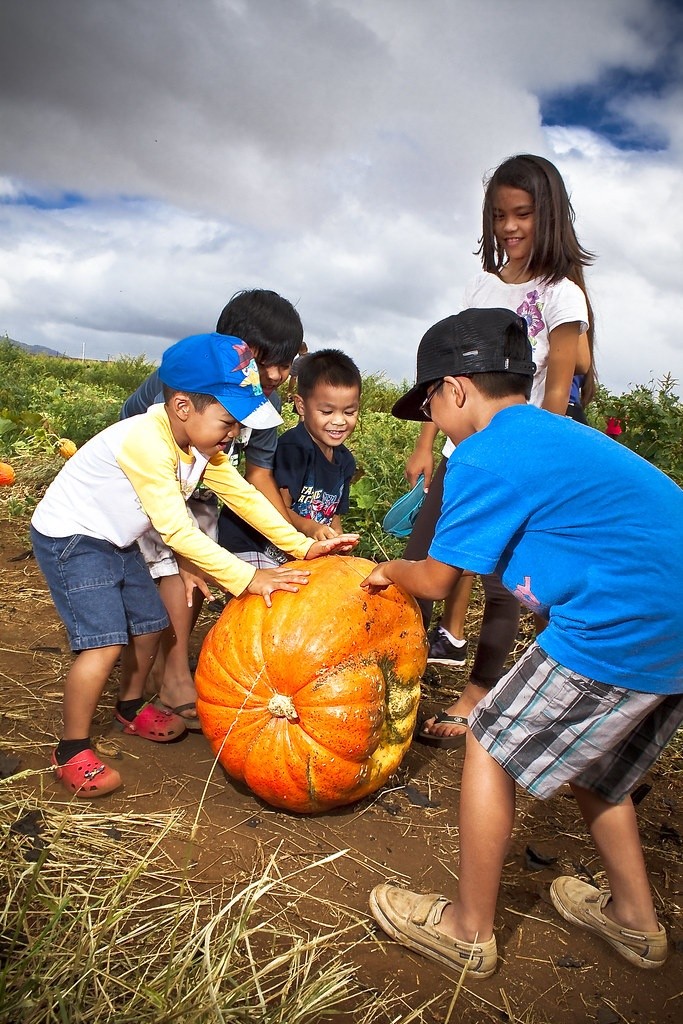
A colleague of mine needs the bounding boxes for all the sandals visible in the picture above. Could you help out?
[51,744,121,798]
[112,702,185,742]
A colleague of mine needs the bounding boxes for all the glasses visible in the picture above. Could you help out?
[419,380,445,418]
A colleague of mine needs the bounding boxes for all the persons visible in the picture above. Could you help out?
[402,152,629,749]
[358,306,682,982]
[28,288,363,801]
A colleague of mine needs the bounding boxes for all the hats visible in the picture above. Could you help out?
[157,332,284,430]
[382,473,427,538]
[391,308,536,421]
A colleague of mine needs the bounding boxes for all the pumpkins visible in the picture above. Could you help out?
[57,438,77,459]
[0,463,16,485]
[194,556,430,813]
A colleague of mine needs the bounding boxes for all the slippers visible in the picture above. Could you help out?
[154,697,201,729]
[144,689,159,701]
[419,710,466,748]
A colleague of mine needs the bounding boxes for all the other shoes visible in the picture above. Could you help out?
[427,622,468,666]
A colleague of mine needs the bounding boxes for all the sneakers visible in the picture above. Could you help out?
[369,884,497,981]
[549,876,668,970]
